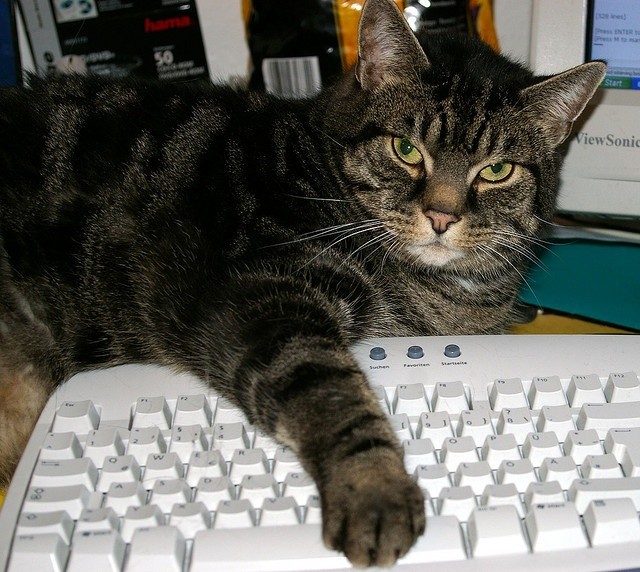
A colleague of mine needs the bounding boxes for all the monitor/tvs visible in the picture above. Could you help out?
[492,0,639,216]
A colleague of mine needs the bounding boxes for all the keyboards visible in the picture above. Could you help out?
[0,333,639,570]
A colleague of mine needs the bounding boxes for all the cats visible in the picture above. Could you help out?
[2,0,610,572]
